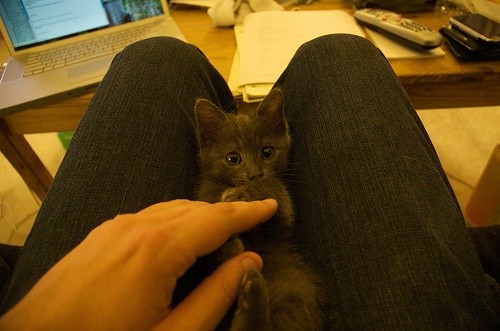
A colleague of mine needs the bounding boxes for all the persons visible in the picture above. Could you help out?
[0,33,500,331]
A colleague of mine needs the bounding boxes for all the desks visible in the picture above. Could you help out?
[0,0,499,209]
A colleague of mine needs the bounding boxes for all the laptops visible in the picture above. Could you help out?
[0,0,189,118]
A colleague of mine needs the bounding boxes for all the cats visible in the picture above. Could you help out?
[192,88,321,331]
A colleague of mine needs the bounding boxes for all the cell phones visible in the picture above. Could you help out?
[440,14,500,62]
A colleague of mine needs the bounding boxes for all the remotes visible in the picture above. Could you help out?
[353,5,443,48]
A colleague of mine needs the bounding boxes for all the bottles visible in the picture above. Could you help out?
[435,2,449,31]
